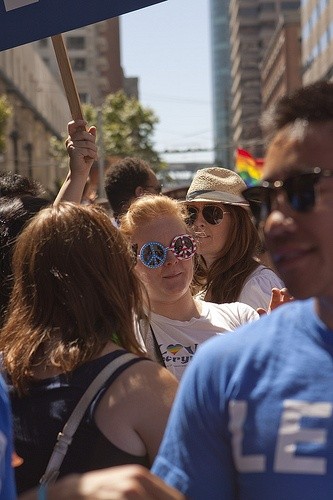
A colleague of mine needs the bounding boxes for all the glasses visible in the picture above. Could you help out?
[127,243,138,271]
[138,184,164,195]
[184,203,231,226]
[239,165,333,221]
[136,233,197,269]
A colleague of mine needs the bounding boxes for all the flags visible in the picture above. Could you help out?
[233,146,265,188]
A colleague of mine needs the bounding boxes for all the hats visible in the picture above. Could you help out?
[174,167,250,206]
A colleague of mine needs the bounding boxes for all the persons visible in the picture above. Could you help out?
[0,77,333,500]
